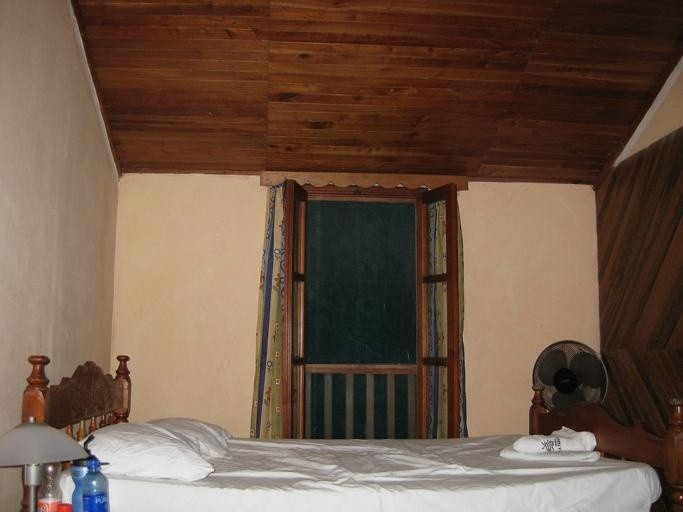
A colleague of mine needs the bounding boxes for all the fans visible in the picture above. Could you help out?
[533,339,610,417]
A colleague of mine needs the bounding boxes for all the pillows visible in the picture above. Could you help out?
[78,417,232,482]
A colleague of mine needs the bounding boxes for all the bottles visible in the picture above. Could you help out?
[37,456,110,512]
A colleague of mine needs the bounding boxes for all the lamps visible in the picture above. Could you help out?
[0,417,91,512]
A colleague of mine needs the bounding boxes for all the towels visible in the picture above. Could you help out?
[498,426,600,462]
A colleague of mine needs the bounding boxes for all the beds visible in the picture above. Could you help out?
[21,352,683,512]
